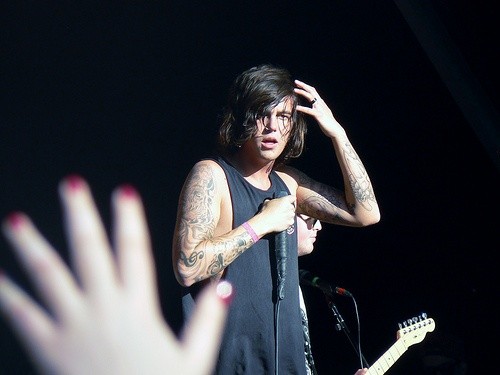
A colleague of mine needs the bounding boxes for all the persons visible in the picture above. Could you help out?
[296,211,369,375]
[171,66,382,375]
[1,176,235,375]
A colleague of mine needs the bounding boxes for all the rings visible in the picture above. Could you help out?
[291,203,295,209]
[310,97,317,106]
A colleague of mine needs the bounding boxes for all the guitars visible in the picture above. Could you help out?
[362,312,436,375]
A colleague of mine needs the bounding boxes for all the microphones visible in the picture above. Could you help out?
[273,191,288,300]
[299,269,350,296]
